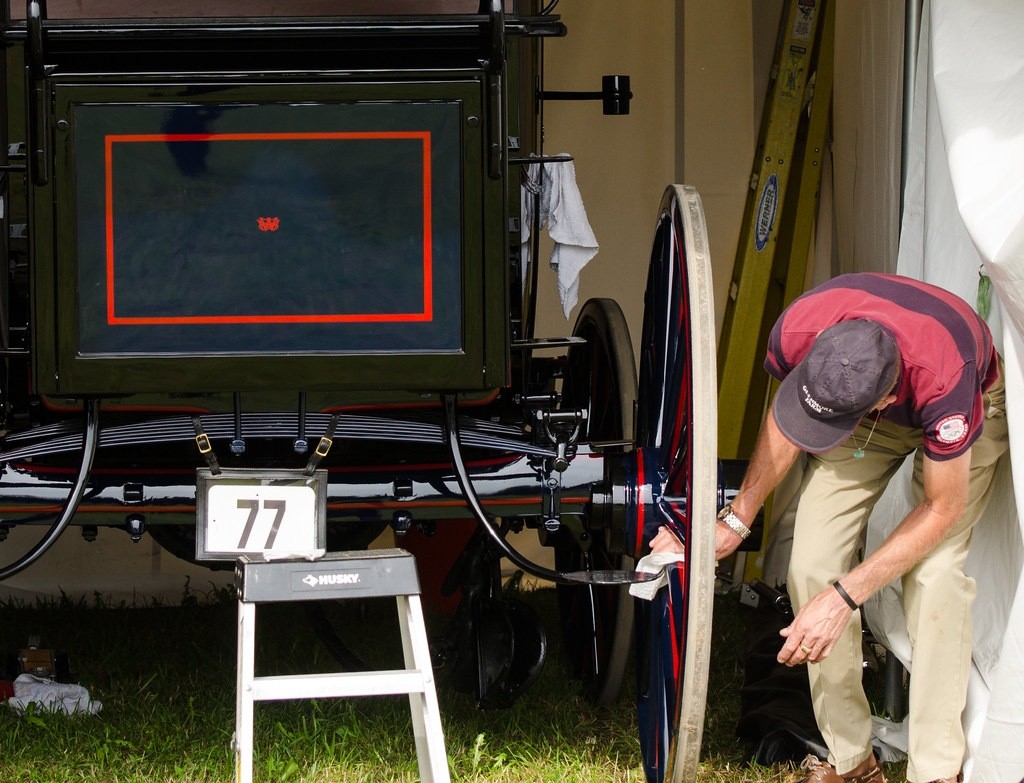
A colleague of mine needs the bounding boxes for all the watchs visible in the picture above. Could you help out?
[717,505,751,539]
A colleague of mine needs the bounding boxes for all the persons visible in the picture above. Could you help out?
[649,270,1009,783]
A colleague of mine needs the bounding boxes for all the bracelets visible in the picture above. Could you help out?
[833,581,860,611]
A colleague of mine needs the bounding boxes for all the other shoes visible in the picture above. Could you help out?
[796,750,884,783]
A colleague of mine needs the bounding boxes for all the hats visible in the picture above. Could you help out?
[772,318,897,451]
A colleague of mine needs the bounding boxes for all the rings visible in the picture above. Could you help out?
[800,643,812,655]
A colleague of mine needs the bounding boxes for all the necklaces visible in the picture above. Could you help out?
[851,410,880,458]
